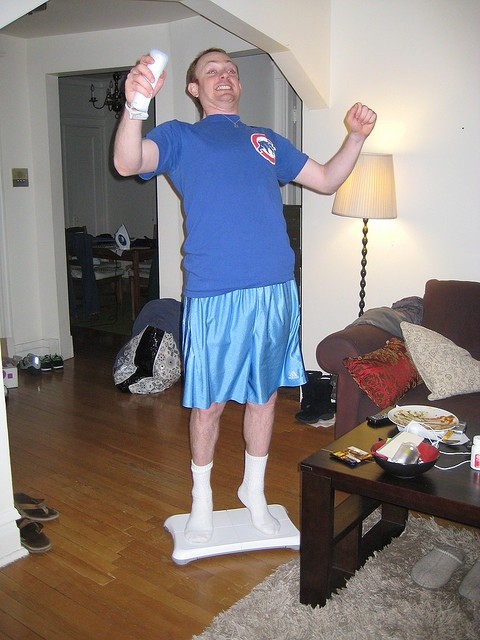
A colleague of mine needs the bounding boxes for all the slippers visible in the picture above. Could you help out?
[14,492,59,521]
[17,518,52,554]
[411,546,463,589]
[459,556,480,600]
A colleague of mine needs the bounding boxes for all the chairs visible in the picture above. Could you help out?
[65,227,121,268]
[65,233,124,320]
[130,248,158,322]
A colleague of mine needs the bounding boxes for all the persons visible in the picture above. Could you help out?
[113,46,377,544]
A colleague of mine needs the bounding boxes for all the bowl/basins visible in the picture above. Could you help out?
[370,437,440,479]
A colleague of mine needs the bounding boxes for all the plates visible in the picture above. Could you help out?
[387,405,459,432]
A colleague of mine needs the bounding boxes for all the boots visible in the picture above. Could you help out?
[301,371,322,410]
[295,380,334,424]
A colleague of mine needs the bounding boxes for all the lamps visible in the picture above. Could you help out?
[330,151,397,318]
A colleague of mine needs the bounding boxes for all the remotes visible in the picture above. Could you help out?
[366,412,388,427]
[131,48,169,113]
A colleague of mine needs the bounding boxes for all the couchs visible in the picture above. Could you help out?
[316,279,480,440]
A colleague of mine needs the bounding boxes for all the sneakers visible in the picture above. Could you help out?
[20,353,40,375]
[39,355,51,371]
[50,353,63,369]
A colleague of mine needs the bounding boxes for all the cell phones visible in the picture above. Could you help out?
[450,420,467,435]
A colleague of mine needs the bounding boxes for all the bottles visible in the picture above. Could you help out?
[470,434,479,471]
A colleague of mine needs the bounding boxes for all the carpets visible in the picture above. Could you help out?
[190,508,480,639]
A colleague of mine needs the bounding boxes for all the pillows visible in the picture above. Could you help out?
[342,336,424,410]
[399,321,480,402]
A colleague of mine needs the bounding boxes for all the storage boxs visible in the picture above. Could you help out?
[0,337,19,390]
